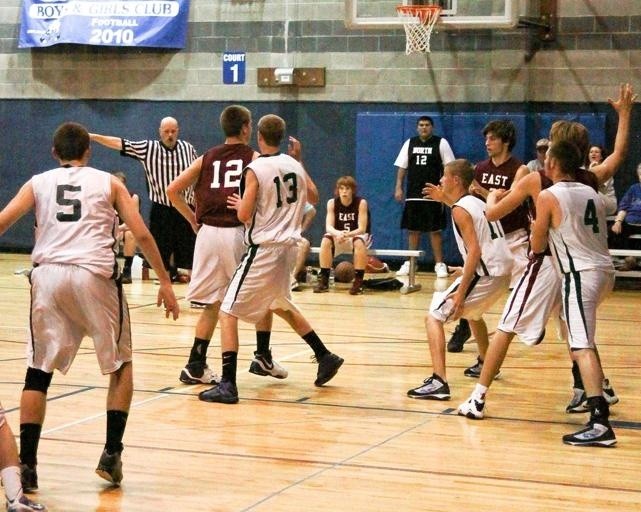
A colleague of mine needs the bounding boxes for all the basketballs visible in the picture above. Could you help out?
[334,260,356,283]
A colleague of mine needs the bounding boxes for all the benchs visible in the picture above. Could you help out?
[117,242,150,281]
[307,248,426,294]
[604,214,641,277]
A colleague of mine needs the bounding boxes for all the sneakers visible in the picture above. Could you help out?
[566,379,618,413]
[5,464,48,512]
[464,356,501,379]
[311,353,344,387]
[249,348,288,379]
[458,398,484,419]
[407,377,450,400]
[563,422,617,447]
[121,266,191,283]
[199,381,238,404]
[313,278,363,295]
[96,449,123,483]
[180,365,222,384]
[435,263,449,278]
[396,261,410,276]
[448,325,471,352]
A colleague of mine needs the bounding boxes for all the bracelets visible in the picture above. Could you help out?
[615,219,622,222]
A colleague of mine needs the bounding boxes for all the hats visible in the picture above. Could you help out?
[536,139,550,149]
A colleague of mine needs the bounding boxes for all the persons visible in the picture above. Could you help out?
[89,117,198,282]
[393,115,457,276]
[1,404,46,512]
[447,119,545,351]
[530,140,619,445]
[527,138,550,207]
[290,201,317,291]
[313,175,371,295]
[112,172,140,283]
[458,83,640,420]
[612,162,640,271]
[199,113,344,403]
[166,104,289,384]
[585,144,617,237]
[1,122,182,491]
[406,160,514,400]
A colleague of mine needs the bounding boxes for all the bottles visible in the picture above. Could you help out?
[328,266,336,289]
[306,266,312,286]
